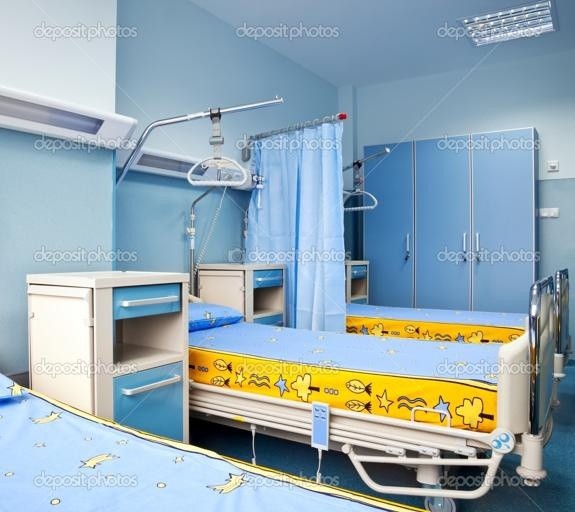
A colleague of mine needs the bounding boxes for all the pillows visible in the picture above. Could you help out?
[187,303,246,331]
[0,372,28,401]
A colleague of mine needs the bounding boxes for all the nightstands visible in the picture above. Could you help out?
[196,262,289,328]
[345,260,369,305]
[26,271,190,446]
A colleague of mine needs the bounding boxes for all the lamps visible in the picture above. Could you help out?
[455,0,561,48]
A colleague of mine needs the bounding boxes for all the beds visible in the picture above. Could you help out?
[0,372,426,512]
[346,268,574,365]
[183,276,565,500]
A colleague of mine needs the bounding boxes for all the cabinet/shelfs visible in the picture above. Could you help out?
[414,127,535,314]
[363,141,415,307]
